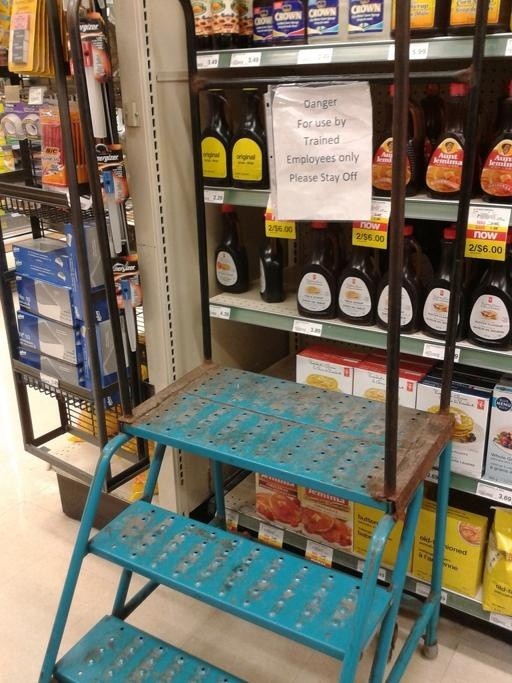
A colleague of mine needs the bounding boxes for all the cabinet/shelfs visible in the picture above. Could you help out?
[191,28,512,634]
[0,0,150,504]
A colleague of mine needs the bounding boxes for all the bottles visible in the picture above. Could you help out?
[215,204,250,294]
[202,88,269,189]
[296,221,512,354]
[259,236,285,301]
[191,0,510,51]
[375,77,512,205]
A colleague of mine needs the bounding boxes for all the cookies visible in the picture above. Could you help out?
[305,374,339,390]
[366,388,386,403]
[427,405,473,439]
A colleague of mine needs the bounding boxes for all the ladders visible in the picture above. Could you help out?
[37,360,455,682]
